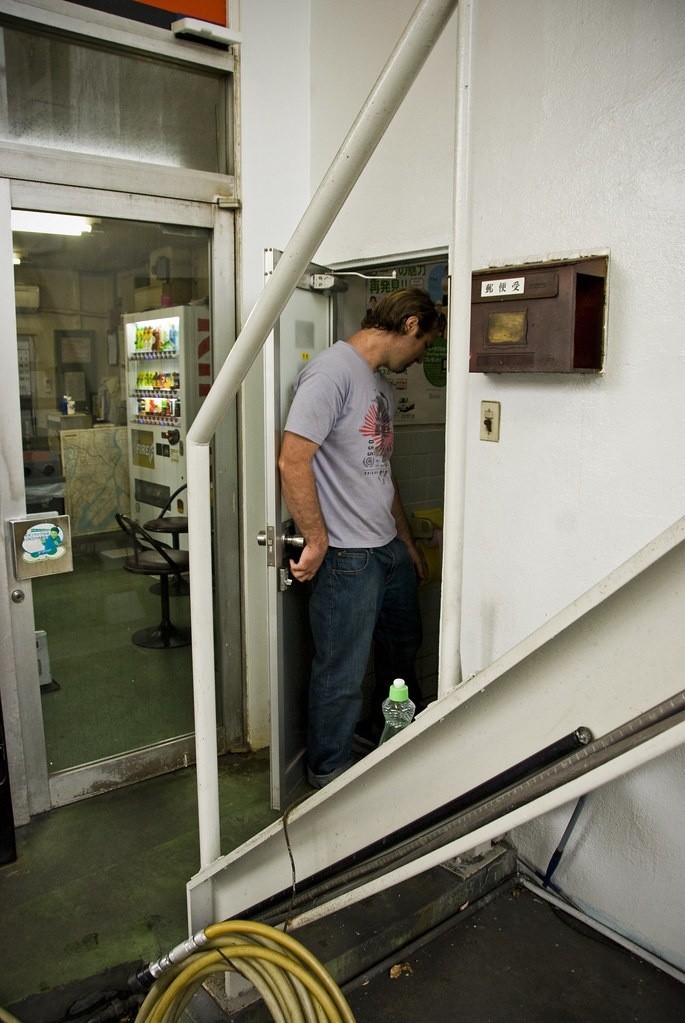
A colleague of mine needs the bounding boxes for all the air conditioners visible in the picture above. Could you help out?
[15,284,40,314]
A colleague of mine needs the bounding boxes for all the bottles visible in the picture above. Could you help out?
[135,325,178,351]
[378,679,416,746]
[136,370,179,389]
[137,396,180,418]
[61,394,76,416]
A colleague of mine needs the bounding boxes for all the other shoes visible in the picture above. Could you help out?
[31,552,39,557]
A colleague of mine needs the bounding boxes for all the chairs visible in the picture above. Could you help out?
[115,512,192,649]
[140,482,190,596]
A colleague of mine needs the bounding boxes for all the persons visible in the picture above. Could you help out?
[275,287,442,798]
[367,295,379,314]
